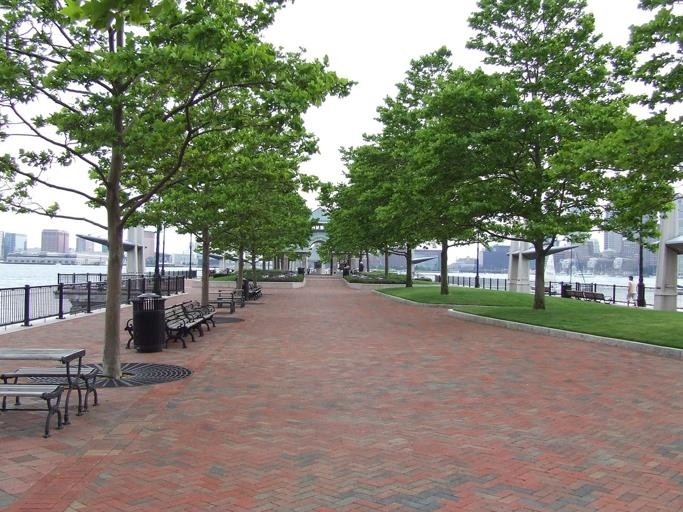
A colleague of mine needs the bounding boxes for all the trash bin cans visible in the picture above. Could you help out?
[297,267,305,278]
[342,267,348,277]
[240,278,248,301]
[131,293,166,353]
[561,284,571,298]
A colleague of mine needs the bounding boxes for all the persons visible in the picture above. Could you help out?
[626,274,638,307]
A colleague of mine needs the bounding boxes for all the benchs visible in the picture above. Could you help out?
[0,347,99,438]
[565,290,614,304]
[124,282,263,350]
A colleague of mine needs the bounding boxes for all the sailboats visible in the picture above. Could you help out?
[530,254,556,292]
